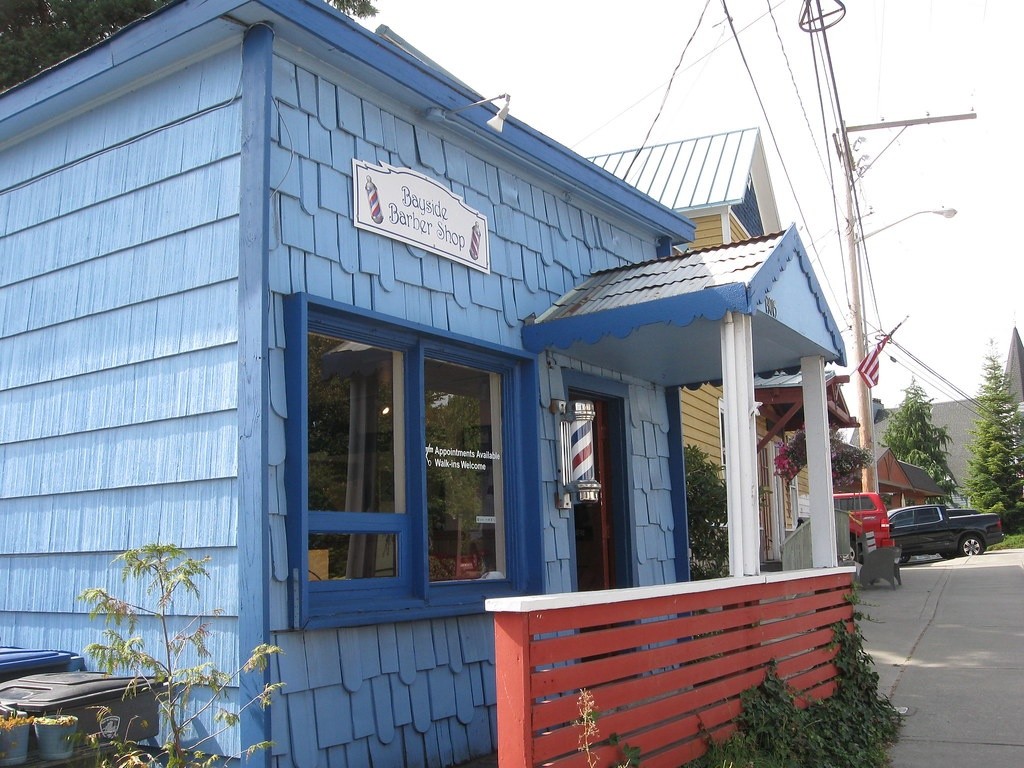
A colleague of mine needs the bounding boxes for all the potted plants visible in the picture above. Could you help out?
[0,715,34,767]
[34,715,78,761]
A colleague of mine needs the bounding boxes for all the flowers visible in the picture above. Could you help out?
[832,447,875,488]
[771,422,846,483]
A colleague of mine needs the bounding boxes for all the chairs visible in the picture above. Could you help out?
[859,530,902,591]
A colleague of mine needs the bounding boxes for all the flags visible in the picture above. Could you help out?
[859,337,889,389]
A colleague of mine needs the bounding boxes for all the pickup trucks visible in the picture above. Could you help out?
[885,504,1005,566]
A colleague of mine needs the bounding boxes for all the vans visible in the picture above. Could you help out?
[833,493,896,565]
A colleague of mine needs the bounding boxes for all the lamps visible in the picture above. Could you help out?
[447,92,512,133]
[550,399,602,510]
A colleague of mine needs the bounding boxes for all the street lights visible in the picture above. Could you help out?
[845,207,961,494]
